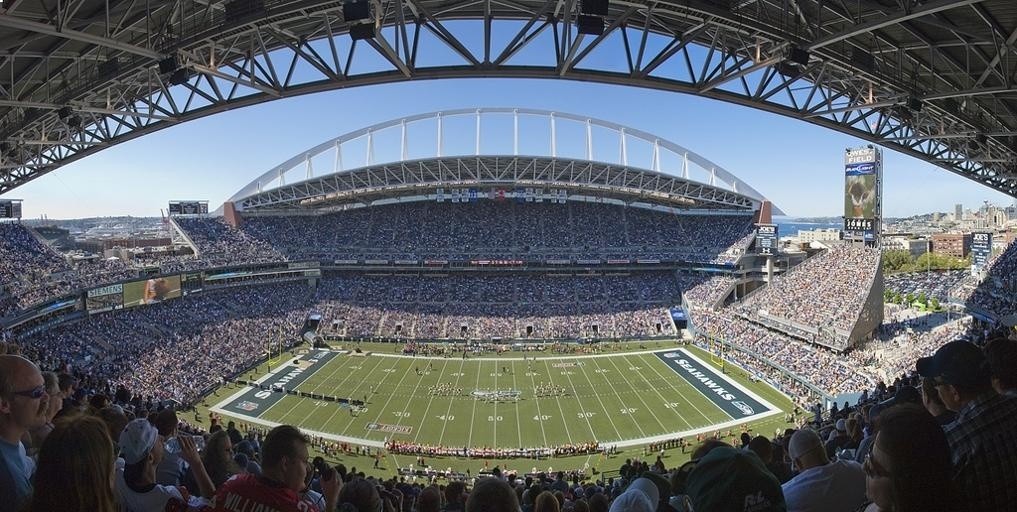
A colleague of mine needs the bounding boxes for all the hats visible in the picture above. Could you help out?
[917,340,992,393]
[119,419,159,466]
[608,475,662,512]
[836,419,846,431]
[678,445,789,511]
[785,428,823,473]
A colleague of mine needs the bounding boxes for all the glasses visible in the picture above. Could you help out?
[13,383,50,399]
[864,440,911,484]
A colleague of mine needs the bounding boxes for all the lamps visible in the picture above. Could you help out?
[772,45,814,80]
[0,141,24,162]
[340,0,382,44]
[153,53,193,88]
[894,96,923,124]
[573,0,615,37]
[964,133,990,152]
[50,102,85,130]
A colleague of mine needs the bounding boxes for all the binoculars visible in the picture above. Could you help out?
[312,456,348,482]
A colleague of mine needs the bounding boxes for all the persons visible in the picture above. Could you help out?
[368,384,374,395]
[745,362,1015,512]
[415,365,419,375]
[2,179,1017,362]
[0,352,265,512]
[502,364,506,375]
[507,365,512,374]
[264,423,743,512]
[349,407,354,416]
[429,361,433,372]
[427,381,572,406]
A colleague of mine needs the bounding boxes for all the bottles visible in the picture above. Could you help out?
[164,436,206,455]
